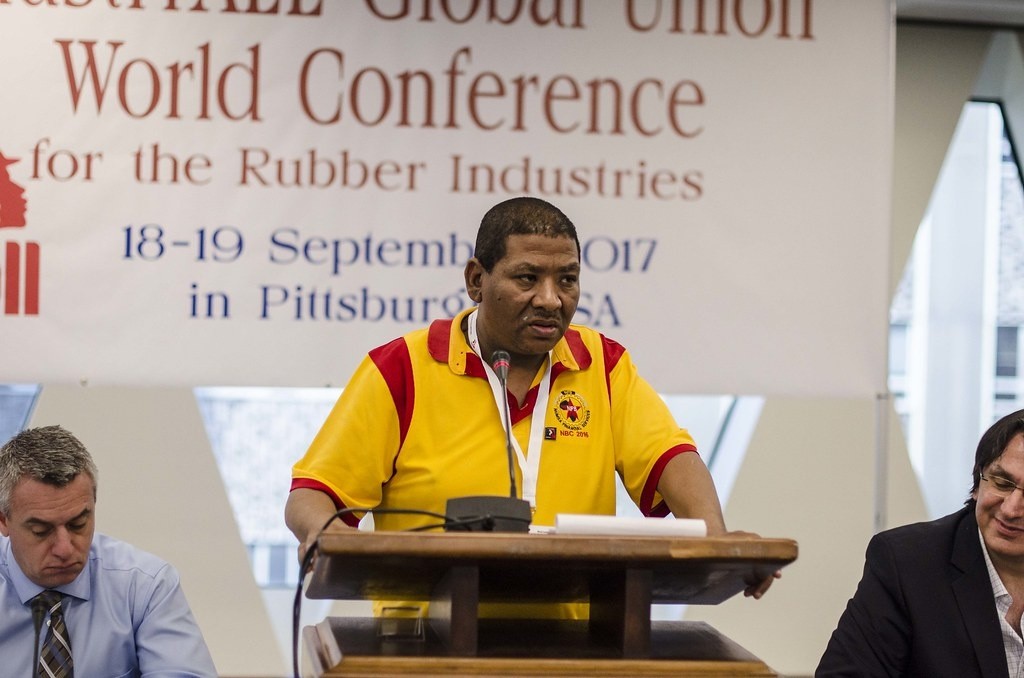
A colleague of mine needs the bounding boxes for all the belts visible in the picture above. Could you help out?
[39,590,75,678]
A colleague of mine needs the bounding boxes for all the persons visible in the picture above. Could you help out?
[814,407,1024,677]
[0,424,220,678]
[281,195,783,623]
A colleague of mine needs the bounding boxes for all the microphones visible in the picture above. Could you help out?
[30,597,49,678]
[491,349,518,498]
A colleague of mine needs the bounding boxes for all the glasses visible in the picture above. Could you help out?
[978,464,1024,498]
